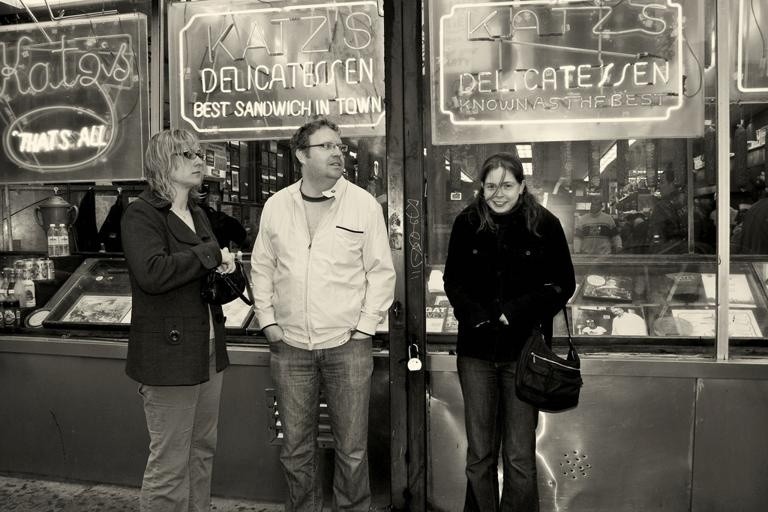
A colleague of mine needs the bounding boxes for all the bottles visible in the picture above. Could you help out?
[45,223,71,258]
[0,267,35,329]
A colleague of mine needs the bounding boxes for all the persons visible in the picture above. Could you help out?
[249,117,396,511]
[442,153,576,512]
[119,129,246,512]
[561,193,767,253]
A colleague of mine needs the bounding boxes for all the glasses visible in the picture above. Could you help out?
[304,143,348,152]
[176,151,206,160]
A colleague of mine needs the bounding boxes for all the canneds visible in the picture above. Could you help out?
[14,258,55,309]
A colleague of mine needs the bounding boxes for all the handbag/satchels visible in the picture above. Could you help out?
[513,327,583,413]
[201,259,255,306]
